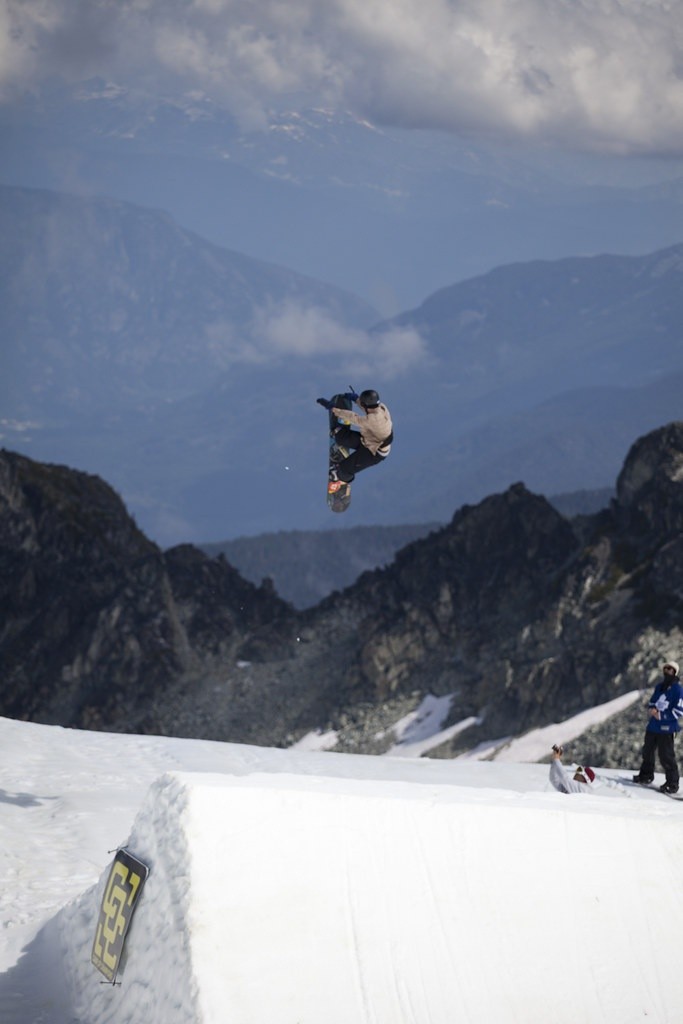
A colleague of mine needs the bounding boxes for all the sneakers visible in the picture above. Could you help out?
[633,773,655,784]
[659,781,680,794]
[329,469,339,482]
[329,425,342,438]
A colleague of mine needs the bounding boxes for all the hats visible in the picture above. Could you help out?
[664,661,680,676]
[584,768,595,782]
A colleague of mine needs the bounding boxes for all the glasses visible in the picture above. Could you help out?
[664,666,673,670]
[576,766,591,783]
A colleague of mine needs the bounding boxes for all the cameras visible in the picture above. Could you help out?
[552,744,563,756]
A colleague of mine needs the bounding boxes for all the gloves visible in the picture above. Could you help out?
[316,397,336,412]
[344,385,359,403]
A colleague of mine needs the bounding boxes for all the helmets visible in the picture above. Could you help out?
[359,390,381,414]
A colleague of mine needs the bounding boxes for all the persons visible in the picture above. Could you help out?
[316,389,395,484]
[632,660,683,794]
[549,750,595,794]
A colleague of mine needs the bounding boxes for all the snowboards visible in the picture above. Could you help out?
[327,389,353,514]
